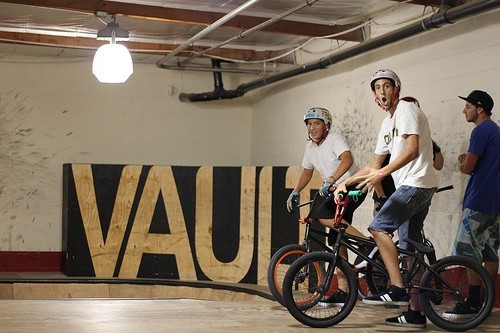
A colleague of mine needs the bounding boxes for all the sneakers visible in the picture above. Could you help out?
[318,288,350,307]
[354,236,379,269]
[443,296,491,319]
[385,304,427,328]
[362,285,411,306]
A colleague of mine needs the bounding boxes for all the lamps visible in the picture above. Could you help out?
[92,16,134,83]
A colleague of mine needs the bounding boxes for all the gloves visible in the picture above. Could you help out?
[286,191,300,212]
[319,180,333,198]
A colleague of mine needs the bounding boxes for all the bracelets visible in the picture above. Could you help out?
[330,176,336,182]
[378,168,386,181]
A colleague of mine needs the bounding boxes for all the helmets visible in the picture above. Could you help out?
[370,69,402,94]
[304,107,332,130]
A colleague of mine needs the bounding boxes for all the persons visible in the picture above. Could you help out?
[371,96,445,306]
[443,89,500,319]
[285,107,367,306]
[329,69,439,327]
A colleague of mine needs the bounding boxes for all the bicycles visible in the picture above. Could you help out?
[266,185,454,310]
[282,189,496,332]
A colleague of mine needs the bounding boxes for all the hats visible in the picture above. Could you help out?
[458,90,494,116]
